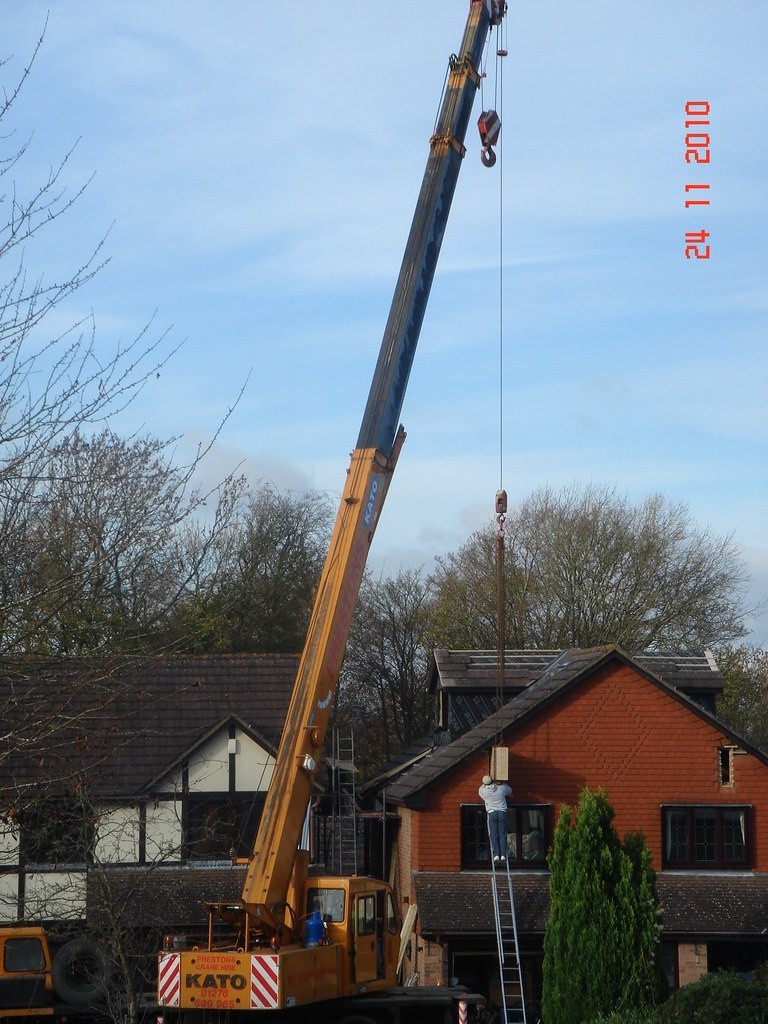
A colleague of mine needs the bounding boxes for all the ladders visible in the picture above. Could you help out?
[491,847,526,1024]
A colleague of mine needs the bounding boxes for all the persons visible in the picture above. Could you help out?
[479,776,512,861]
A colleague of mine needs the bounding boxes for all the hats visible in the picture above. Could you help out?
[482,776,492,784]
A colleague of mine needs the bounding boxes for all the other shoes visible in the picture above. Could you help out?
[500,856,507,861]
[494,856,500,861]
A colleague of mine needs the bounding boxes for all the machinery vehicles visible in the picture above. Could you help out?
[137,1,508,1024]
[0,928,56,1024]
